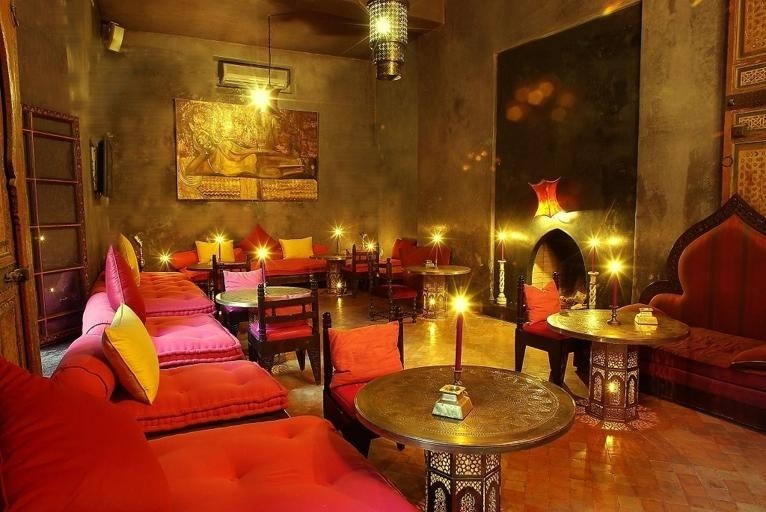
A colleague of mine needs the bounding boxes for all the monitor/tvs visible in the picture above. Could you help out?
[97,138,113,198]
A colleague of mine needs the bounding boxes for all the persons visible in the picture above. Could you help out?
[182,128,315,178]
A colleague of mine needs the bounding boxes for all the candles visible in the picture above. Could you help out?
[499,238,506,260]
[611,269,622,316]
[590,244,599,273]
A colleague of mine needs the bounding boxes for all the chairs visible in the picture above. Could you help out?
[515,272,590,388]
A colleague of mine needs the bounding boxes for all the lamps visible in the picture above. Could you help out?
[528,175,565,222]
[365,1,410,85]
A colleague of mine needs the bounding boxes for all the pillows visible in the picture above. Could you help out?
[522,280,562,325]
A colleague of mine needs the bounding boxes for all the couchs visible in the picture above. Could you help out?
[2,223,453,510]
[641,188,766,435]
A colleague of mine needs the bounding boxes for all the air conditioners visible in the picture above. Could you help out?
[216,58,290,90]
